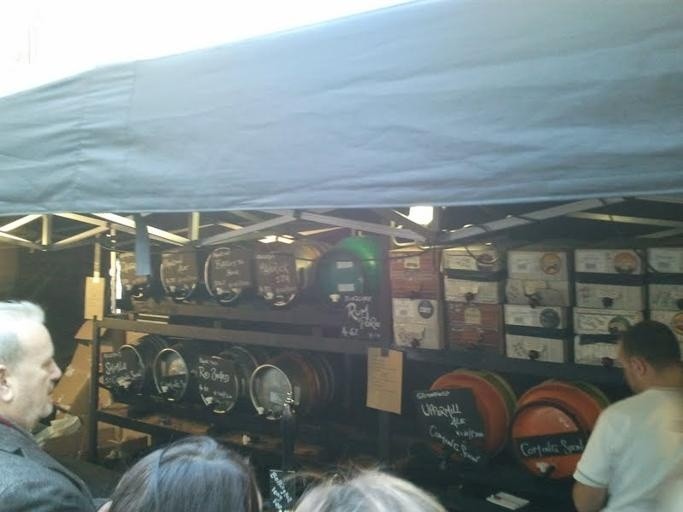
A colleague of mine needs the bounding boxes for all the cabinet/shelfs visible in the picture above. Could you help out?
[70,314,370,480]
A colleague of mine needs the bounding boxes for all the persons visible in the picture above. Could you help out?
[572,322,683,512]
[292,465,448,512]
[0,298,98,512]
[108,434,262,512]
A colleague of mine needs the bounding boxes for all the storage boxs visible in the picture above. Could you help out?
[384,240,682,377]
